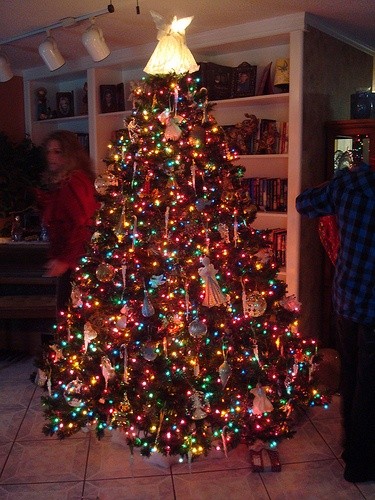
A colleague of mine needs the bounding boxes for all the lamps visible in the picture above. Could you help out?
[82,15,112,62]
[40,28,67,73]
[0,52,15,84]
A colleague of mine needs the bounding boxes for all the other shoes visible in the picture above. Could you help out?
[343,466,375,483]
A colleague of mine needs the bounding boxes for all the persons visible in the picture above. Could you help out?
[294,163,375,483]
[35,131,101,324]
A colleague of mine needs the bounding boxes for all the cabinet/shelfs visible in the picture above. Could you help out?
[21,11,304,339]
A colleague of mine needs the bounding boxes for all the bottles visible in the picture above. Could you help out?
[13,216,22,241]
[40,228,48,242]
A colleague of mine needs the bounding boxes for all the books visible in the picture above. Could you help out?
[243,176,288,213]
[259,228,287,267]
[223,119,289,155]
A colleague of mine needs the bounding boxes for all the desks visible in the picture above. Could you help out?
[0,234,70,353]
